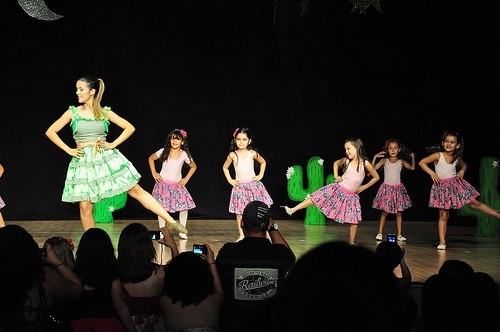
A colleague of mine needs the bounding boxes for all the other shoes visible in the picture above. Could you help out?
[165,220,188,234]
[285,205,293,215]
[397,235,406,241]
[235,235,244,242]
[437,244,446,250]
[160,231,165,239]
[376,234,382,240]
[179,232,188,239]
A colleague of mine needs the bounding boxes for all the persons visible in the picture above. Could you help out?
[0,164,5,228]
[371,139,415,241]
[223,127,274,242]
[45,75,188,234]
[148,129,197,240]
[285,138,380,246]
[418,130,500,249]
[0,201,500,332]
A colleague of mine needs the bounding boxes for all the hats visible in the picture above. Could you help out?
[243,201,270,229]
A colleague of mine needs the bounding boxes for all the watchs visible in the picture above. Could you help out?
[269,222,279,232]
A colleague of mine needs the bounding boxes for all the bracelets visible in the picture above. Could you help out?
[56,263,66,266]
[208,262,216,265]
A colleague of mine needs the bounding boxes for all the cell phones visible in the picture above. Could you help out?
[193,244,205,255]
[387,234,396,242]
[149,231,165,239]
